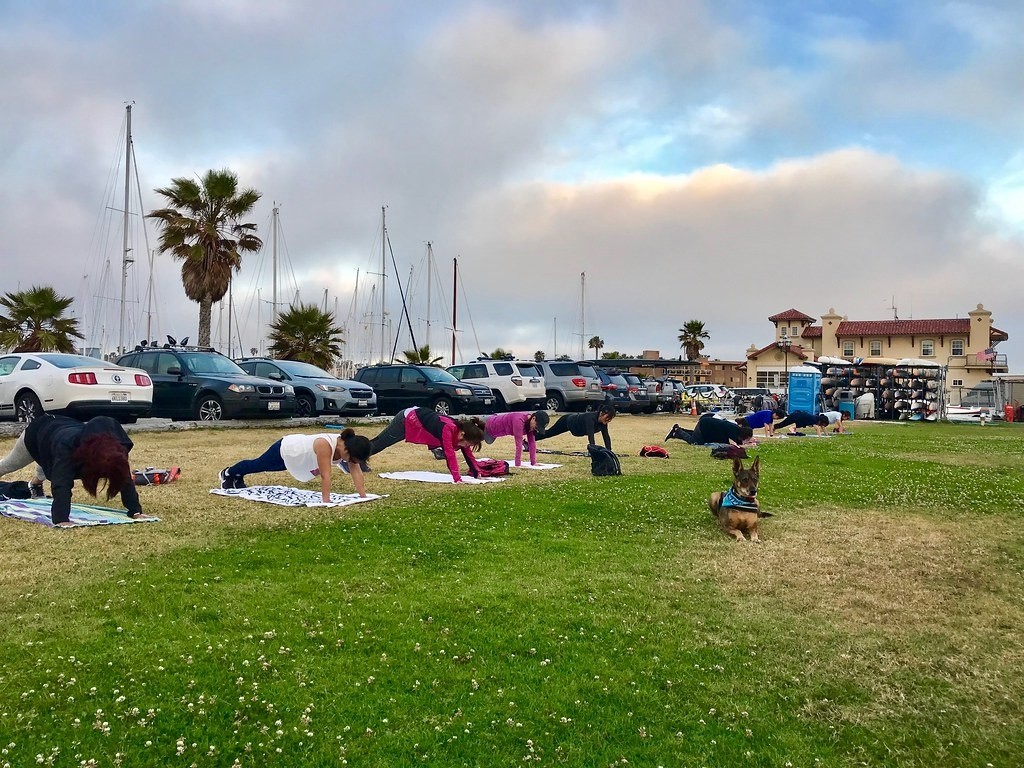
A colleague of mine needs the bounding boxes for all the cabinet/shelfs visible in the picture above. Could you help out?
[823,363,948,423]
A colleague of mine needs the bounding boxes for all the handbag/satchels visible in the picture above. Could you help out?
[640,446,668,459]
[712,445,748,460]
[132,466,171,485]
[0,481,31,501]
[469,459,509,476]
[167,467,181,482]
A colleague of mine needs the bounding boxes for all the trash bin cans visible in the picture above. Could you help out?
[839,391,855,421]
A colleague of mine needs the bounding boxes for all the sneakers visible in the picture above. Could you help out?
[219,467,234,490]
[28,480,45,499]
[233,475,246,488]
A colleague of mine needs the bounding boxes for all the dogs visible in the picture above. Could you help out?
[708,456,775,544]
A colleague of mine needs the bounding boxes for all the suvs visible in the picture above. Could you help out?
[352,363,497,417]
[223,356,377,419]
[432,356,548,413]
[531,359,605,413]
[109,343,298,422]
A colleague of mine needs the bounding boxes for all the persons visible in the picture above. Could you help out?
[338,407,494,483]
[219,427,370,503]
[0,413,150,526]
[665,393,852,447]
[432,411,550,467]
[523,404,616,451]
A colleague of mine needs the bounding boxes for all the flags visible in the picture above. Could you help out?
[978,348,995,359]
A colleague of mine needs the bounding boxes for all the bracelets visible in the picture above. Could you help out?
[134,512,141,515]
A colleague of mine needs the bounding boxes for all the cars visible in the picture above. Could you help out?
[0,352,155,424]
[594,368,782,414]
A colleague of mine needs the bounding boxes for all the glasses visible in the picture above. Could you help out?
[349,453,356,464]
[847,416,849,420]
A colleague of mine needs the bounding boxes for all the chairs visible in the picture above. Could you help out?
[159,362,175,374]
[469,368,476,377]
[196,360,211,371]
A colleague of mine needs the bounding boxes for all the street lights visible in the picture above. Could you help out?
[777,334,793,417]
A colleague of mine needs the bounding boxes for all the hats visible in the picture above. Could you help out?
[536,411,549,434]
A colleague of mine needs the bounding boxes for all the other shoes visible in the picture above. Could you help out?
[521,439,529,451]
[672,424,679,429]
[665,428,675,442]
[432,447,445,460]
[360,462,371,472]
[337,459,350,474]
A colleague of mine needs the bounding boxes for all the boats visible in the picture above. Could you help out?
[801,354,1006,423]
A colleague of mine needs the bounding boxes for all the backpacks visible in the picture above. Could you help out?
[587,444,620,475]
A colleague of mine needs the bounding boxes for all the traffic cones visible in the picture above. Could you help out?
[689,398,698,415]
